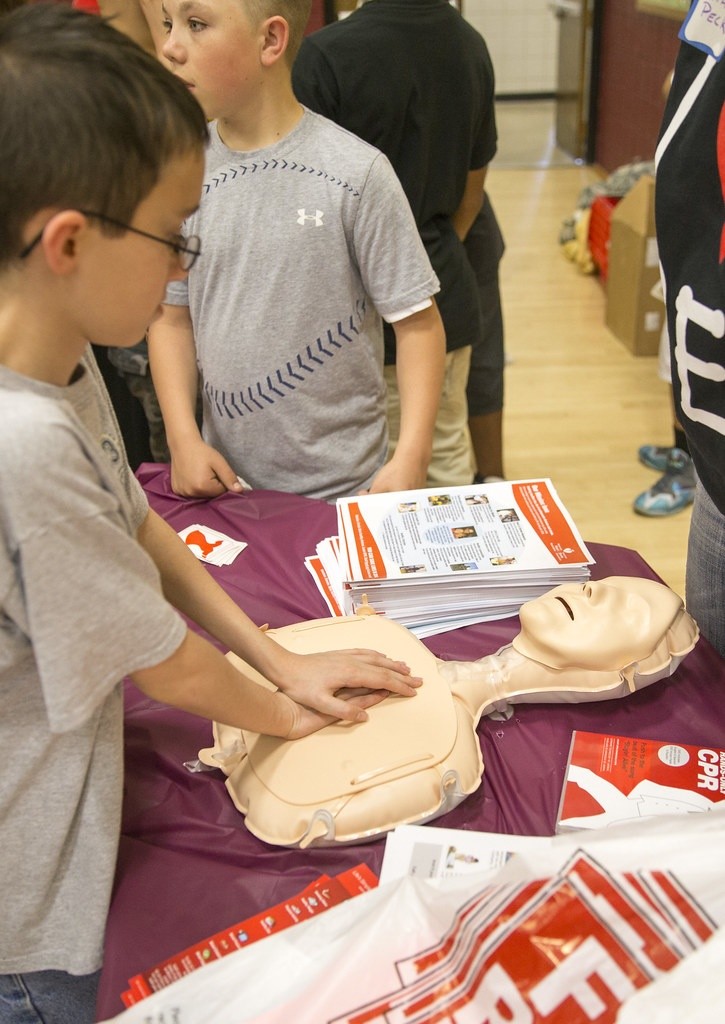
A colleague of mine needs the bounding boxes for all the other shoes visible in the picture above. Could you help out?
[472,473,498,484]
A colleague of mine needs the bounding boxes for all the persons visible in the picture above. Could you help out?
[634,256,695,519]
[70,0,158,64]
[290,0,498,486]
[198,576,699,849]
[1,0,424,1022]
[148,0,446,501]
[450,192,506,488]
[655,0,724,658]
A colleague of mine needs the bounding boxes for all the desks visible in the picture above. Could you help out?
[92,463,725,1024]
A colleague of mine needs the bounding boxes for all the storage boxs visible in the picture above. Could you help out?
[589,173,666,354]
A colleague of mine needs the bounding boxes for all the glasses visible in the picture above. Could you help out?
[19,207,203,271]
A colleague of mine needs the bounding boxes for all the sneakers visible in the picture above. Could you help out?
[638,444,692,471]
[634,468,697,517]
